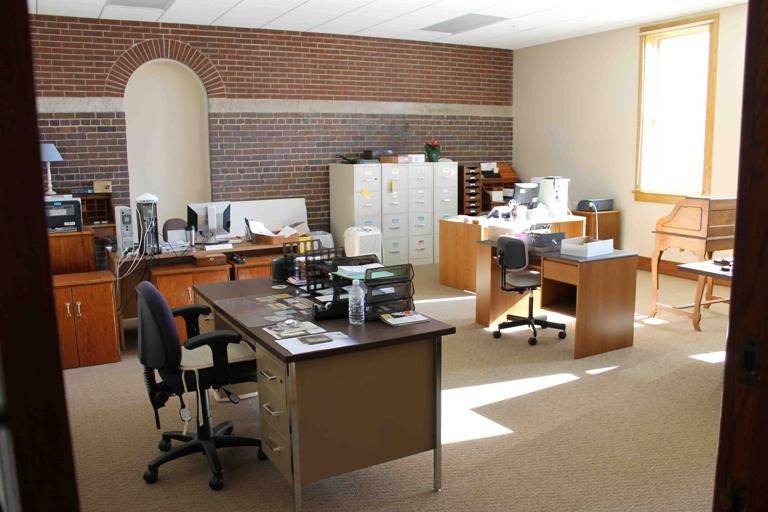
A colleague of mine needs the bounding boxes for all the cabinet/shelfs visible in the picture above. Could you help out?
[229,253,285,280]
[460,162,523,216]
[409,163,434,266]
[150,260,231,347]
[328,163,383,265]
[51,269,121,370]
[382,163,408,267]
[434,161,458,265]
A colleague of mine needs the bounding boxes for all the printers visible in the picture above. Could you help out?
[576,199,613,211]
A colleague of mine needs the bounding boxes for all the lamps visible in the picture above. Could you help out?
[40,143,64,194]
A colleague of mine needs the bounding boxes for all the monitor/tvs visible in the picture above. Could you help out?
[513,183,540,209]
[187,201,231,245]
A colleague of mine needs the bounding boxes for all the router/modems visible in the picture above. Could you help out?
[136,193,159,204]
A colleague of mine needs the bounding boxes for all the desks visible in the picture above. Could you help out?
[676,256,734,280]
[437,215,588,296]
[646,195,737,332]
[475,239,638,361]
[571,209,620,250]
[105,234,307,355]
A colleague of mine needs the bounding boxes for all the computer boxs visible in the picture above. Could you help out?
[115,206,134,256]
[136,204,160,254]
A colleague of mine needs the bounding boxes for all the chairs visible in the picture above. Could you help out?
[134,280,269,491]
[492,236,567,347]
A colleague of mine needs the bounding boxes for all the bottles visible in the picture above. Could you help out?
[185,225,194,246]
[347,278,366,326]
